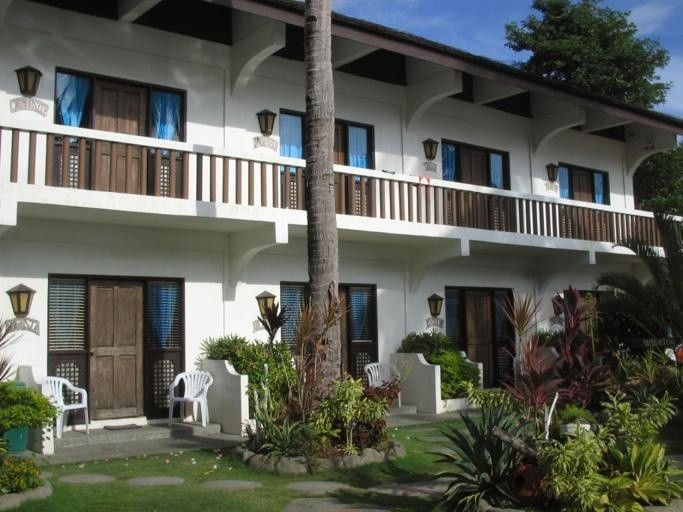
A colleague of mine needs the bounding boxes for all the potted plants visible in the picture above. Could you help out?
[0,377,64,456]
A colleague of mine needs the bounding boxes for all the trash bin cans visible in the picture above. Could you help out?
[0,381,27,452]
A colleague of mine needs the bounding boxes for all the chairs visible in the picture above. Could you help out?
[166,369,215,428]
[362,360,403,410]
[38,375,89,440]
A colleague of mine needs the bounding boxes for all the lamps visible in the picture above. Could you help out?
[254,289,276,320]
[4,283,35,319]
[13,65,43,97]
[426,291,444,319]
[544,162,560,182]
[255,109,277,138]
[420,137,439,161]
